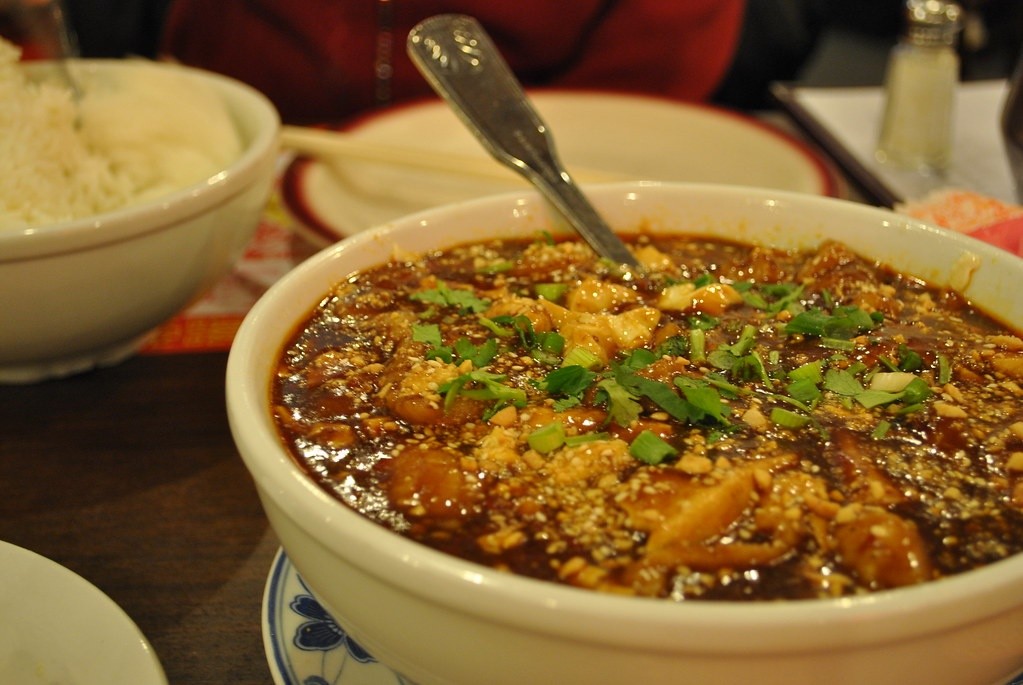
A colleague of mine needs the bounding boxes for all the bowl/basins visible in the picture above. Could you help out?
[226,182,1023,685]
[0,60,281,384]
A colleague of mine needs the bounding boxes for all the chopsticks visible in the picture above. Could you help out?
[280,126,643,185]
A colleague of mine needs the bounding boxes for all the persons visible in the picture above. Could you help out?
[153,1,745,128]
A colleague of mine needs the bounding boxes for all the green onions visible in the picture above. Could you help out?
[529,279,927,468]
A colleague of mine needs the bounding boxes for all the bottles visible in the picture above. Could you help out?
[877,0,962,174]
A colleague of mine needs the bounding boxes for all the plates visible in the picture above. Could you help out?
[0,540,169,685]
[280,88,848,248]
[260,545,1023,685]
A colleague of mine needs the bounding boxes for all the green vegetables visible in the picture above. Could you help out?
[408,228,952,428]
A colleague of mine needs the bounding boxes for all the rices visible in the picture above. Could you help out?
[1,37,241,237]
